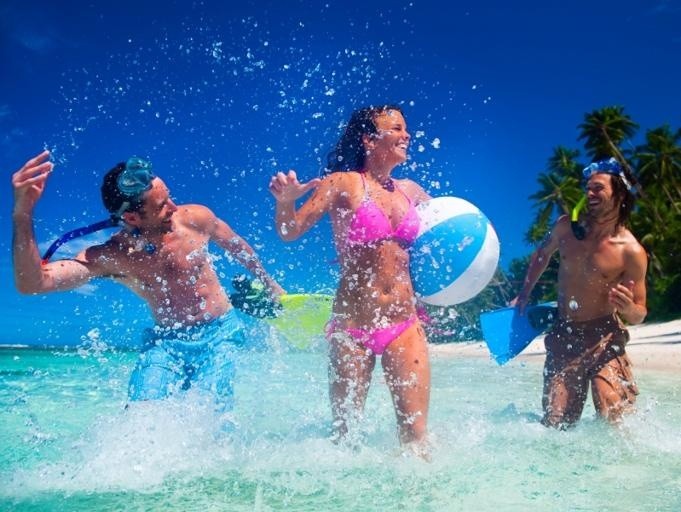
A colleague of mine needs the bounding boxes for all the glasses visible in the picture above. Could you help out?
[582,158,635,194]
[114,158,154,218]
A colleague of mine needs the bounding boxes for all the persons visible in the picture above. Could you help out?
[11,149,286,432]
[268,103,432,460]
[507,171,648,429]
